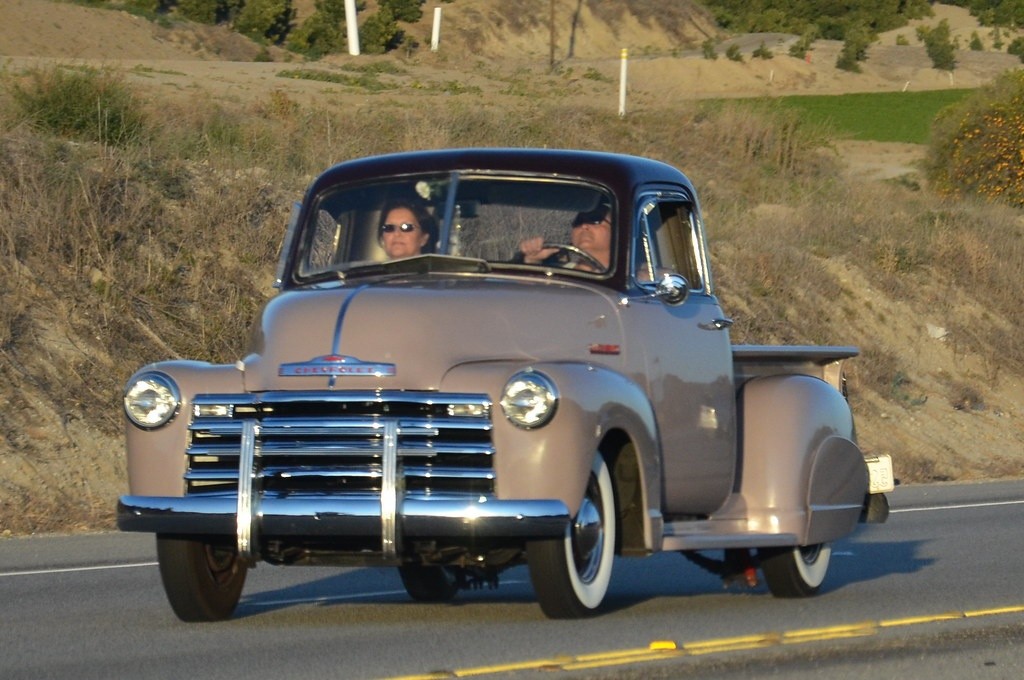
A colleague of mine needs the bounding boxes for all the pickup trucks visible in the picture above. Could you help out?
[117,147,902,622]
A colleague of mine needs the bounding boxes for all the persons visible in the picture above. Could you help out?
[518,198,676,287]
[376,192,440,259]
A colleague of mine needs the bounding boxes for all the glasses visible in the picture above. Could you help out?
[572,212,612,227]
[381,223,422,233]
